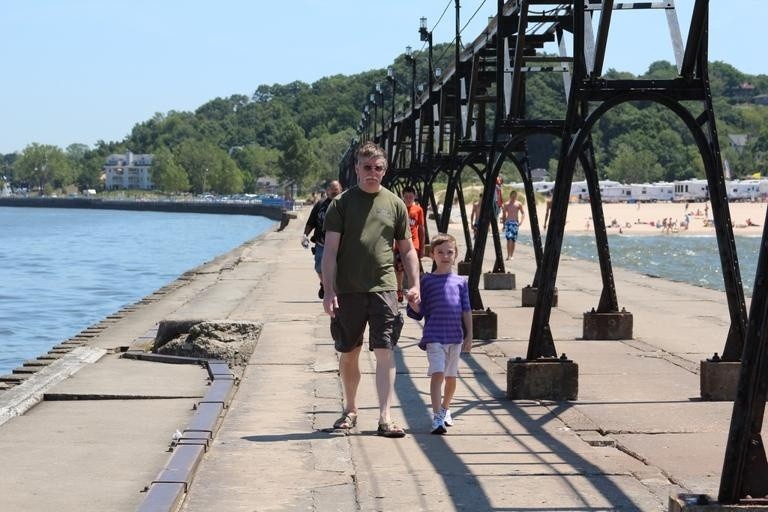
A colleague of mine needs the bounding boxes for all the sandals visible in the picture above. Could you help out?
[378,421,405,436]
[397,286,403,302]
[334,411,358,429]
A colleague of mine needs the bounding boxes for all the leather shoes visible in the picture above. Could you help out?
[318,282,324,298]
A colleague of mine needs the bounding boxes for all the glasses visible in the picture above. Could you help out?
[364,165,383,172]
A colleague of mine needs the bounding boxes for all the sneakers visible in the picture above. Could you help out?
[440,405,454,426]
[431,417,447,433]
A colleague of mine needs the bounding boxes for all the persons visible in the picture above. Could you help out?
[393,186,427,303]
[320,190,327,201]
[542,188,555,230]
[321,143,422,437]
[502,190,525,261]
[605,202,753,236]
[300,179,343,300]
[406,232,474,434]
[302,190,316,208]
[470,192,484,248]
